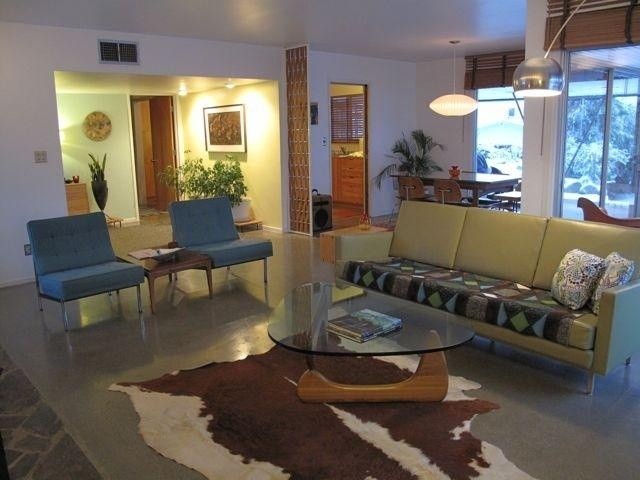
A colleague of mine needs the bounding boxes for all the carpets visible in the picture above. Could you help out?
[106,326,530,479]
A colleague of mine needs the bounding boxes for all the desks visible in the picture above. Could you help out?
[397,171,521,207]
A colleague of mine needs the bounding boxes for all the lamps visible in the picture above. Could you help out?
[428,40,480,118]
[512,0,584,98]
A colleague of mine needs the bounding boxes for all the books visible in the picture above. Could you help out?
[325,308,403,344]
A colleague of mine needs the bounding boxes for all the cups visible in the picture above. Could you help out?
[73,176,79,183]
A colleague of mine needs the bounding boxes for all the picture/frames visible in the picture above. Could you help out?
[202,103,248,153]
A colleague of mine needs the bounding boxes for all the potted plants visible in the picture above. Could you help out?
[156,149,252,222]
[87,150,109,220]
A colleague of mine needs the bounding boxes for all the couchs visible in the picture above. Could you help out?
[334,199,640,396]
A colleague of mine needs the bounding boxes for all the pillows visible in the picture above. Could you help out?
[550,248,608,311]
[585,250,635,316]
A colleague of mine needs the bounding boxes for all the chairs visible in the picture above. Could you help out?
[25,210,146,335]
[433,179,468,205]
[395,175,432,202]
[491,191,521,210]
[168,195,273,283]
[575,196,640,228]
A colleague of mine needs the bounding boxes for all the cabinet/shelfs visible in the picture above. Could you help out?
[66,183,89,214]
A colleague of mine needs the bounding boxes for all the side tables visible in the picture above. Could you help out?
[114,241,221,314]
